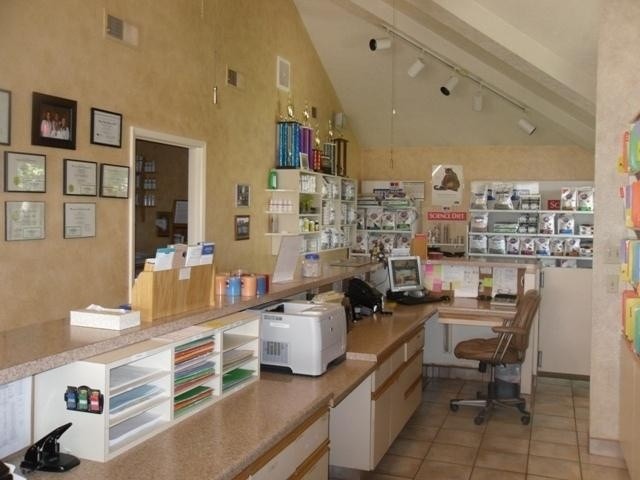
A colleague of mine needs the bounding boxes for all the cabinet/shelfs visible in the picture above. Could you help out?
[465,180,596,269]
[619,116,639,480]
[131,260,215,322]
[33,310,262,462]
[349,180,425,259]
[264,169,357,255]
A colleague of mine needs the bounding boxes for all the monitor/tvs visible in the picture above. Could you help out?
[388,256,423,297]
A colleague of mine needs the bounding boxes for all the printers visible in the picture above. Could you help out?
[249,298,347,377]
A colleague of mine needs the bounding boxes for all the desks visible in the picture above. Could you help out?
[260,359,377,408]
[0,379,333,480]
[438,297,517,326]
[329,298,438,471]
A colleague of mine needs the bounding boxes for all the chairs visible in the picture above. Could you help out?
[449,289,542,425]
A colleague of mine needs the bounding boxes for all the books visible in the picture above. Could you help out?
[173,338,214,419]
[224,351,255,390]
[110,365,165,416]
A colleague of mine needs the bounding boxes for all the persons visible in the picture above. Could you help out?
[42,111,69,139]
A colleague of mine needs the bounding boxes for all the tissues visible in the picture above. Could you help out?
[70,304,141,330]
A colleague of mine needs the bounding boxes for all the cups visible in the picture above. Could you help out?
[214,269,270,297]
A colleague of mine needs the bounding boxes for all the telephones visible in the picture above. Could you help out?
[348,278,393,314]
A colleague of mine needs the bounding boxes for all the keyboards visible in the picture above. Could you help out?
[398,295,440,305]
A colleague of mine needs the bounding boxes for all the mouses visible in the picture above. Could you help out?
[441,296,450,301]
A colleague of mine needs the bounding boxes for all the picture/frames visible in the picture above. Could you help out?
[63,159,98,196]
[5,201,45,241]
[31,91,78,150]
[63,202,95,239]
[0,89,11,145]
[234,184,250,208]
[234,215,250,241]
[4,151,46,193]
[99,163,130,199]
[90,107,123,148]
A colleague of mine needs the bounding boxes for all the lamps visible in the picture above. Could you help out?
[369,39,391,51]
[472,91,483,112]
[517,118,537,136]
[440,76,459,97]
[407,57,425,78]
